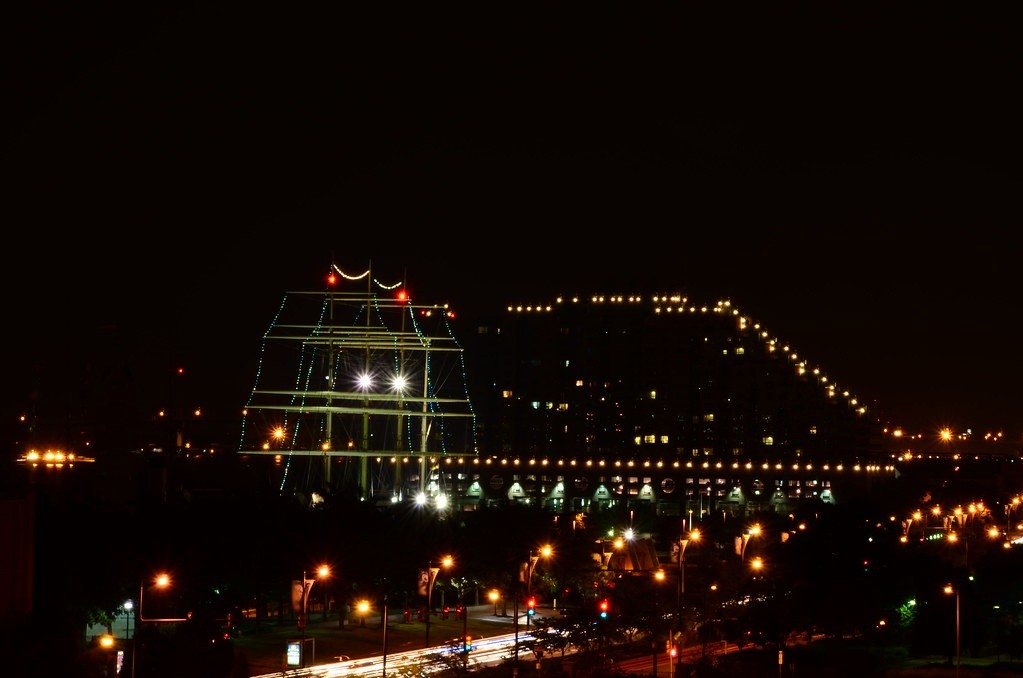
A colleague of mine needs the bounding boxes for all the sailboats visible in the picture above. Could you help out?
[236,251,478,551]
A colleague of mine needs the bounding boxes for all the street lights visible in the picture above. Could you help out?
[678,528,701,616]
[601,539,623,570]
[488,588,518,666]
[426,554,453,648]
[738,522,763,600]
[139,570,170,678]
[528,544,554,630]
[300,561,329,669]
[354,598,387,678]
[944,582,960,678]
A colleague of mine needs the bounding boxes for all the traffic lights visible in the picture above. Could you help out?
[456,606,462,621]
[526,597,535,615]
[417,609,424,621]
[465,635,471,651]
[442,605,448,619]
[600,601,608,619]
[403,610,411,622]
